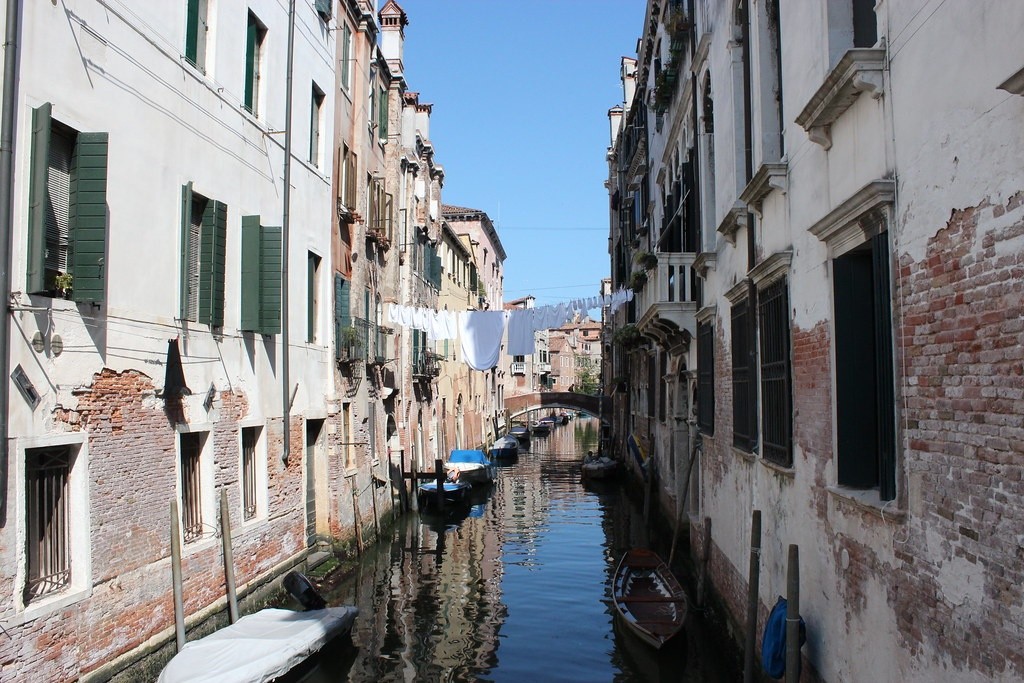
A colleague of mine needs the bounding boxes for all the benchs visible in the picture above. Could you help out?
[617,597,684,602]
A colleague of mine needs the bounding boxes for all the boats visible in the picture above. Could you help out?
[489,434,520,458]
[155,607,359,683]
[542,411,576,424]
[418,472,473,504]
[531,422,551,432]
[581,456,625,479]
[598,549,689,650]
[508,425,530,441]
[444,449,497,484]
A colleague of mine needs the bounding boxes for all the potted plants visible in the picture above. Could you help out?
[340,327,359,356]
[54,273,73,299]
[634,250,657,270]
[625,271,646,294]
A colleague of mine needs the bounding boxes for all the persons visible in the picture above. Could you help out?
[584,451,594,464]
[568,384,574,392]
